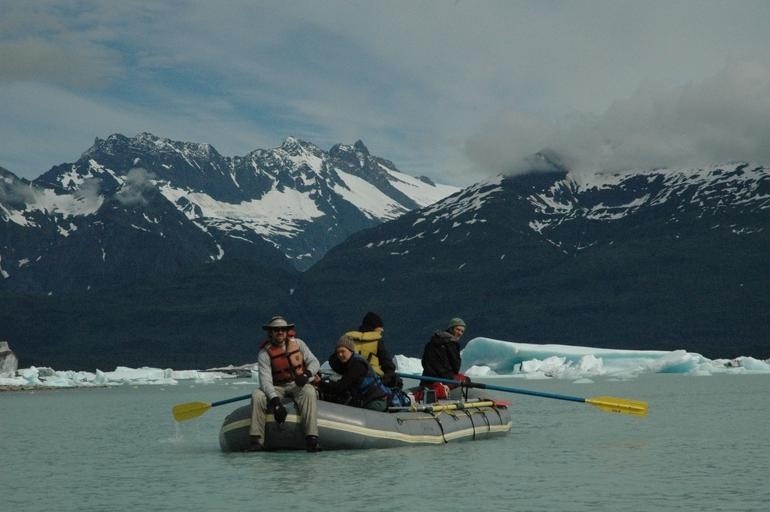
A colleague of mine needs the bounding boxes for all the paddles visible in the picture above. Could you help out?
[173,394,251,422]
[396,372,648,416]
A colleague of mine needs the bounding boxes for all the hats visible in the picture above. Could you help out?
[336,335,354,352]
[363,312,384,329]
[263,316,296,331]
[448,318,465,328]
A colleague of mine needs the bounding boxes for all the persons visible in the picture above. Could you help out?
[344,311,403,389]
[286,326,297,339]
[313,334,390,413]
[248,315,324,454]
[419,317,472,392]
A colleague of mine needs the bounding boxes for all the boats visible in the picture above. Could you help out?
[219,397,514,452]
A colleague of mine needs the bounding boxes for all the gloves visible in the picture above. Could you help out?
[271,397,287,423]
[296,370,312,387]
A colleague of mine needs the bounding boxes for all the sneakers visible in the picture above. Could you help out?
[305,437,321,452]
[245,436,263,451]
